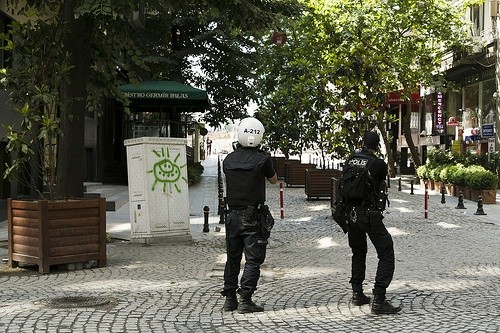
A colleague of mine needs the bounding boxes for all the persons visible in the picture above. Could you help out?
[221,117,277,313]
[340,131,401,314]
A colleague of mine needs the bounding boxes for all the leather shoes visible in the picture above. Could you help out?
[352,290,370,306]
[372,301,401,314]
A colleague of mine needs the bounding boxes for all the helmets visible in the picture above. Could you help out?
[238,117,265,147]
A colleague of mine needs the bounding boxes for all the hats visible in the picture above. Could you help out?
[364,131,380,150]
[333,205,348,234]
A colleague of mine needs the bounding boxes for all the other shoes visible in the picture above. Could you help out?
[224,296,239,311]
[237,298,264,314]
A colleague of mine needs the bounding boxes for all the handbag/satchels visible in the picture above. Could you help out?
[376,180,390,211]
[260,205,275,239]
[339,164,376,202]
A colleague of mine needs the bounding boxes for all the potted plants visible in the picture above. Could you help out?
[0,19,107,276]
[254,65,377,201]
[415,164,498,204]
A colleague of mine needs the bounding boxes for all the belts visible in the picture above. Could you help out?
[229,209,245,216]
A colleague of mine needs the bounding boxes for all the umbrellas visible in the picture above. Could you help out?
[117,80,212,137]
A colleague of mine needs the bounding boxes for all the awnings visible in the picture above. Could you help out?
[338,87,420,111]
